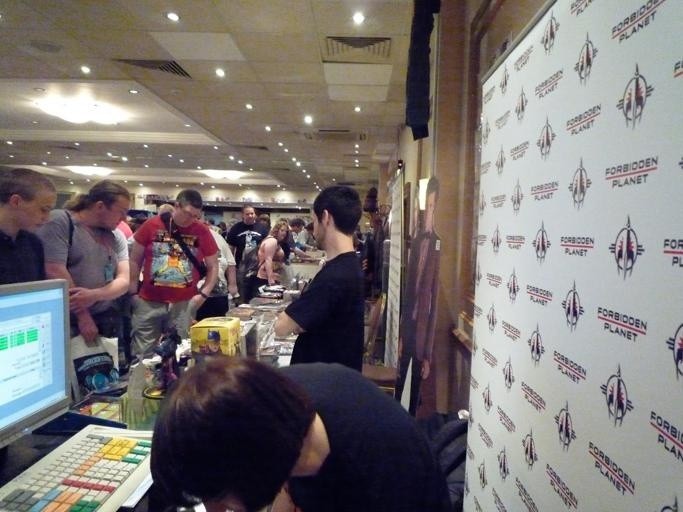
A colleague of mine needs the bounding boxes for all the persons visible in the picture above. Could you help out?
[149,357,454,512]
[391,177,441,417]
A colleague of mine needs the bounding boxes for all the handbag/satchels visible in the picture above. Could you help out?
[197,266,220,297]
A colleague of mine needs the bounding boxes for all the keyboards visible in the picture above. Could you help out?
[0,423,155,512]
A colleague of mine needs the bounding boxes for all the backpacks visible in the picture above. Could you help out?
[244,234,279,279]
[430,418,469,505]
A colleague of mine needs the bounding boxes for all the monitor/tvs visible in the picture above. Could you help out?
[0,279,72,451]
